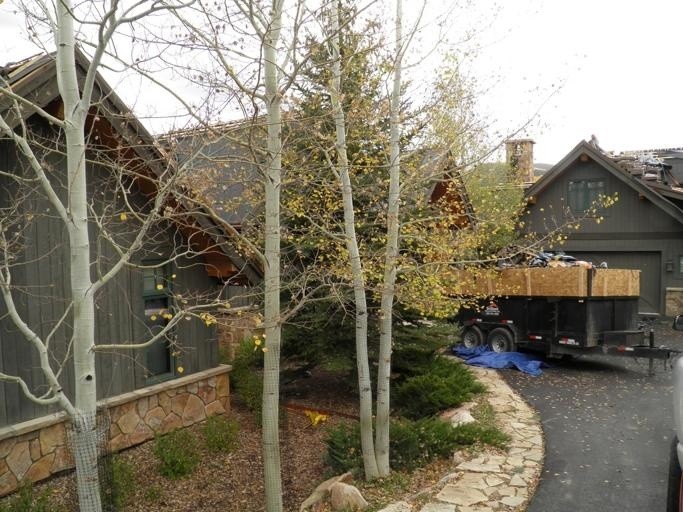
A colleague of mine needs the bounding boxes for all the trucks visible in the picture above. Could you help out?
[420,256,678,355]
